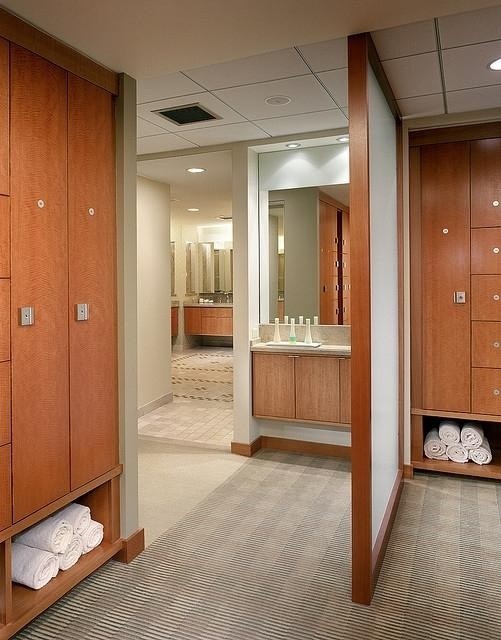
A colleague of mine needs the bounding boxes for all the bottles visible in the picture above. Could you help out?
[274,317,282,343]
[299,315,304,325]
[283,316,289,324]
[290,318,298,346]
[305,319,313,346]
[313,316,320,326]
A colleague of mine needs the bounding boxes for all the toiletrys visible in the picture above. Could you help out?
[298,315,303,324]
[313,315,319,324]
[289,317,296,344]
[273,317,281,343]
[284,315,289,323]
[304,318,313,344]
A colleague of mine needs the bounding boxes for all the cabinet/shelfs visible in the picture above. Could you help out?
[409,120,501,479]
[278,299,285,321]
[319,199,351,325]
[251,352,352,428]
[1,3,122,640]
[184,308,233,337]
[171,307,178,336]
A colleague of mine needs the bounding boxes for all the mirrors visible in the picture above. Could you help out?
[278,254,285,298]
[258,144,350,325]
[198,226,233,293]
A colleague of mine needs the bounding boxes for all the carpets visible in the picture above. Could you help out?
[172,354,233,402]
[8,448,501,640]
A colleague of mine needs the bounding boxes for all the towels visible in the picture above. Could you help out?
[424,421,492,465]
[11,503,103,590]
